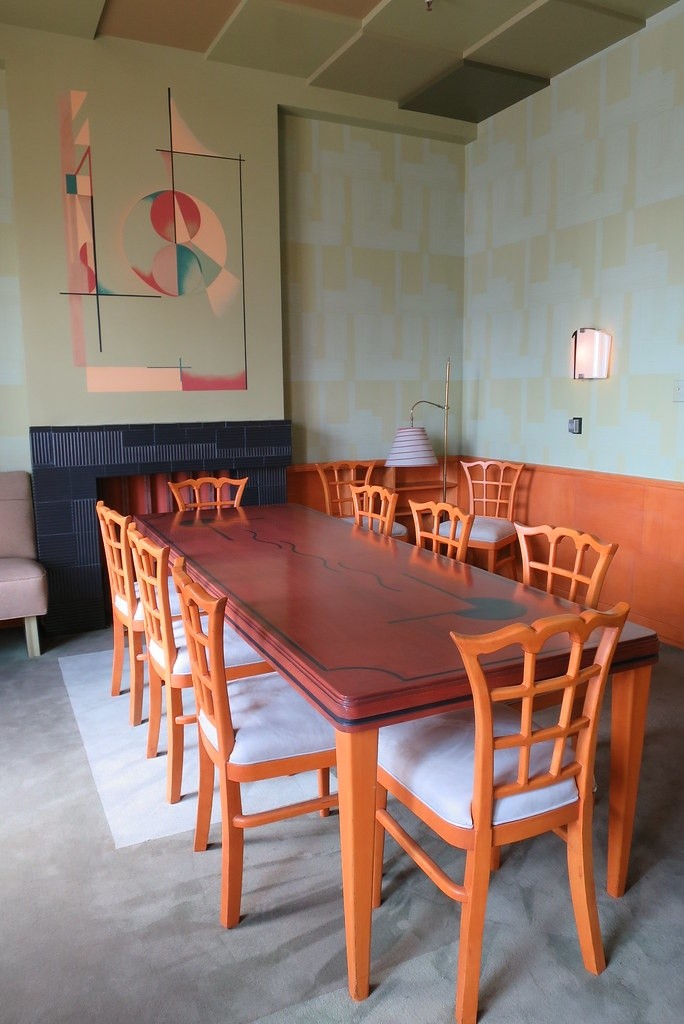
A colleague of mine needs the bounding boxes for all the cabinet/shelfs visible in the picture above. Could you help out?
[382,455,460,521]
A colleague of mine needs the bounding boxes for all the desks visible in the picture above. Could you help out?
[129,499,662,1002]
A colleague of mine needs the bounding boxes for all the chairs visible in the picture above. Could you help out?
[94,457,633,1024]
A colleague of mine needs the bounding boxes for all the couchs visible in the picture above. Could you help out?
[0,471,49,658]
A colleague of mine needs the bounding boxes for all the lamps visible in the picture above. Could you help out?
[570,327,612,379]
[384,358,451,522]
